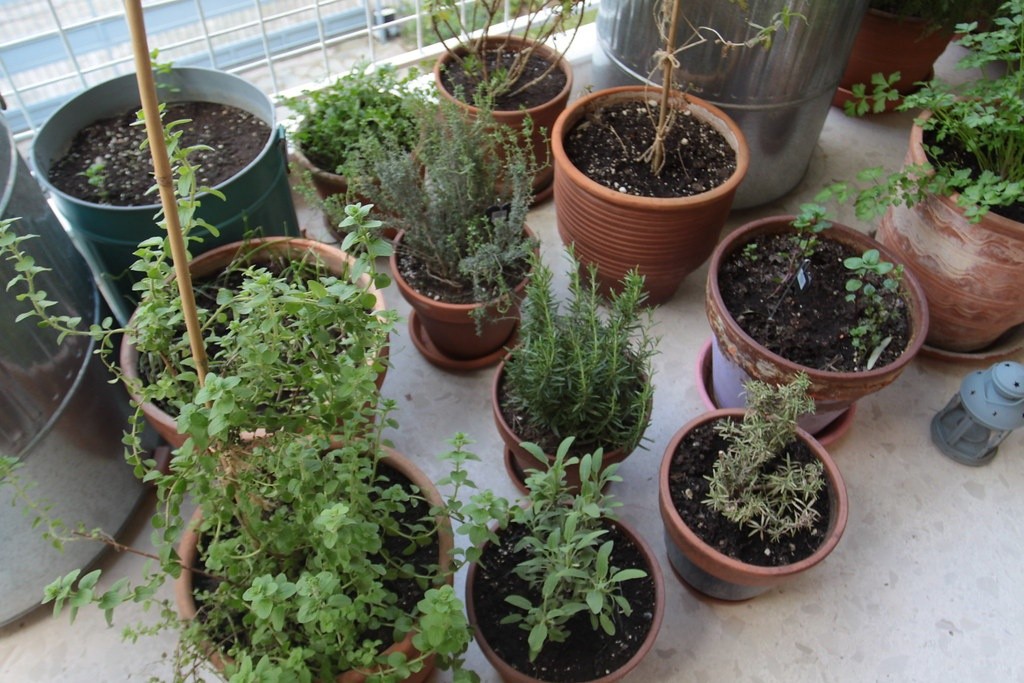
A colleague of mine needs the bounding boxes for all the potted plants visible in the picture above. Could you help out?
[812,0,1024,362]
[549,0,751,307]
[657,367,850,605]
[0,35,542,683]
[464,437,665,683]
[693,178,932,446]
[489,225,666,510]
[830,0,962,115]
[424,0,586,208]
